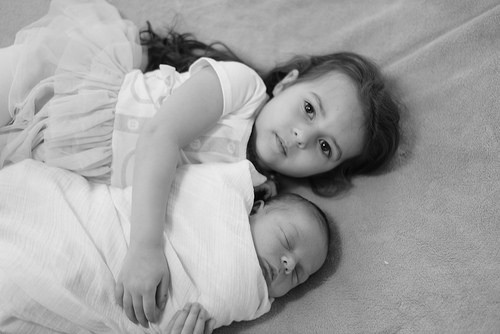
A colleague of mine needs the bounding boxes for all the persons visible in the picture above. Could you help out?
[0,160,330,334]
[0,1,399,334]
